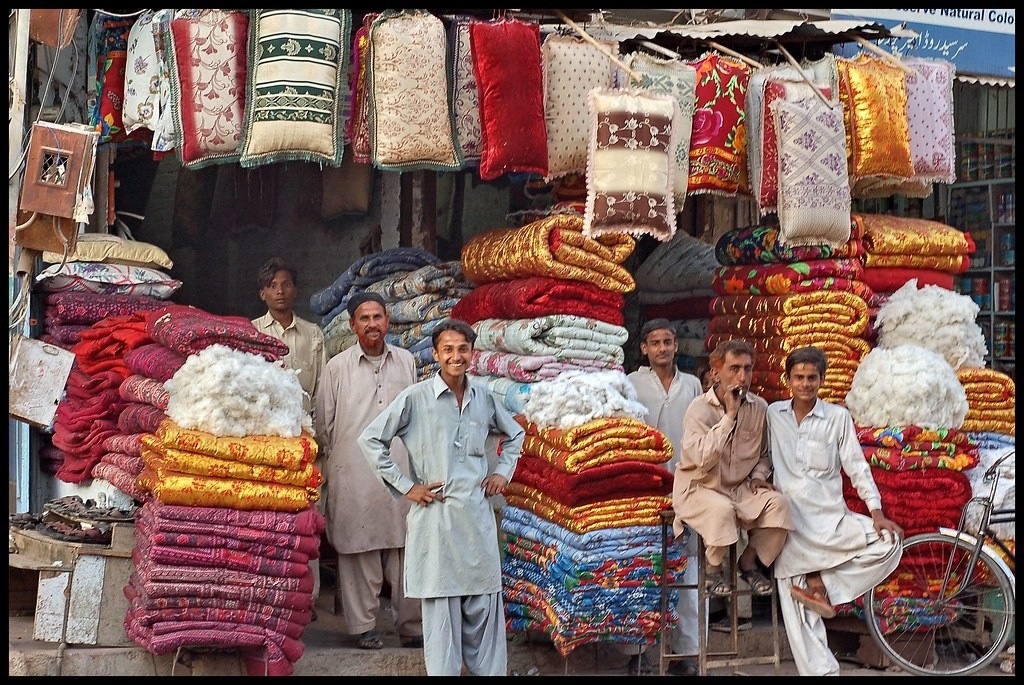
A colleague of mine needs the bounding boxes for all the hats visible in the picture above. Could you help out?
[346,292,386,317]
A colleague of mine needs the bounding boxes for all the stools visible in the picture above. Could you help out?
[659,510,780,676]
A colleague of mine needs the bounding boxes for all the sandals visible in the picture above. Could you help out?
[738,556,774,595]
[705,570,733,595]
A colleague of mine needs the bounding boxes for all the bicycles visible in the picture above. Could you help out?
[864,450,1015,676]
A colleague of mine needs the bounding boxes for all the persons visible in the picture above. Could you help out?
[356,318,526,676]
[313,292,424,649]
[250,261,330,420]
[622,317,905,677]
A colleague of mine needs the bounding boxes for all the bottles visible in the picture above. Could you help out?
[910,198,920,218]
[903,195,909,218]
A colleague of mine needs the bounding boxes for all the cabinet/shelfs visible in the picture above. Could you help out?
[849,176,1016,387]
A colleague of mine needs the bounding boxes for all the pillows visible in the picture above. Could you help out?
[758,74,832,218]
[235,9,352,171]
[745,62,814,205]
[89,9,150,147]
[164,9,250,170]
[122,8,160,135]
[353,12,372,164]
[617,51,696,215]
[367,9,465,172]
[348,10,366,141]
[835,52,957,197]
[151,9,176,161]
[766,97,851,247]
[469,15,548,179]
[443,14,482,170]
[684,50,751,197]
[170,150,376,250]
[542,33,619,184]
[581,87,677,243]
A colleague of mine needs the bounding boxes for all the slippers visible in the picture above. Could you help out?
[358,631,384,649]
[792,585,836,619]
[400,633,424,647]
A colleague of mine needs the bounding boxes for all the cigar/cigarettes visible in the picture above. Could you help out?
[738,388,743,395]
